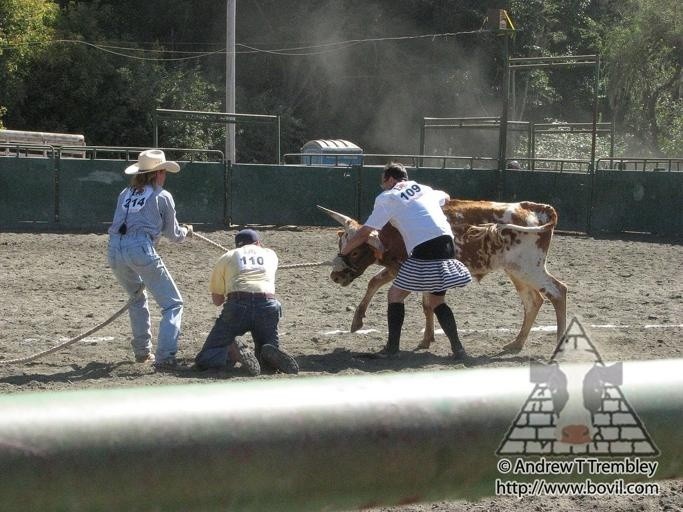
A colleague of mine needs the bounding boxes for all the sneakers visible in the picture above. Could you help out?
[136,353,155,363]
[261,343,299,375]
[228,336,260,376]
[372,345,400,358]
[448,348,467,361]
[155,364,191,373]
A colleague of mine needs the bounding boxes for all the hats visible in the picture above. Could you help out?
[235,229,258,242]
[124,149,180,175]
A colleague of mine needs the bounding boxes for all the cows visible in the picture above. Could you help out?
[316,197,570,354]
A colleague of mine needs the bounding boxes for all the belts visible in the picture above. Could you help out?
[228,291,275,300]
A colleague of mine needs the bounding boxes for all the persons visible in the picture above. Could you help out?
[195,230,299,374]
[506,158,522,169]
[106,150,193,372]
[341,162,471,362]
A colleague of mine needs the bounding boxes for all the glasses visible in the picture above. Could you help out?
[379,179,390,190]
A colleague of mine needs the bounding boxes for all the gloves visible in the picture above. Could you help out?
[181,224,194,239]
[332,257,347,272]
